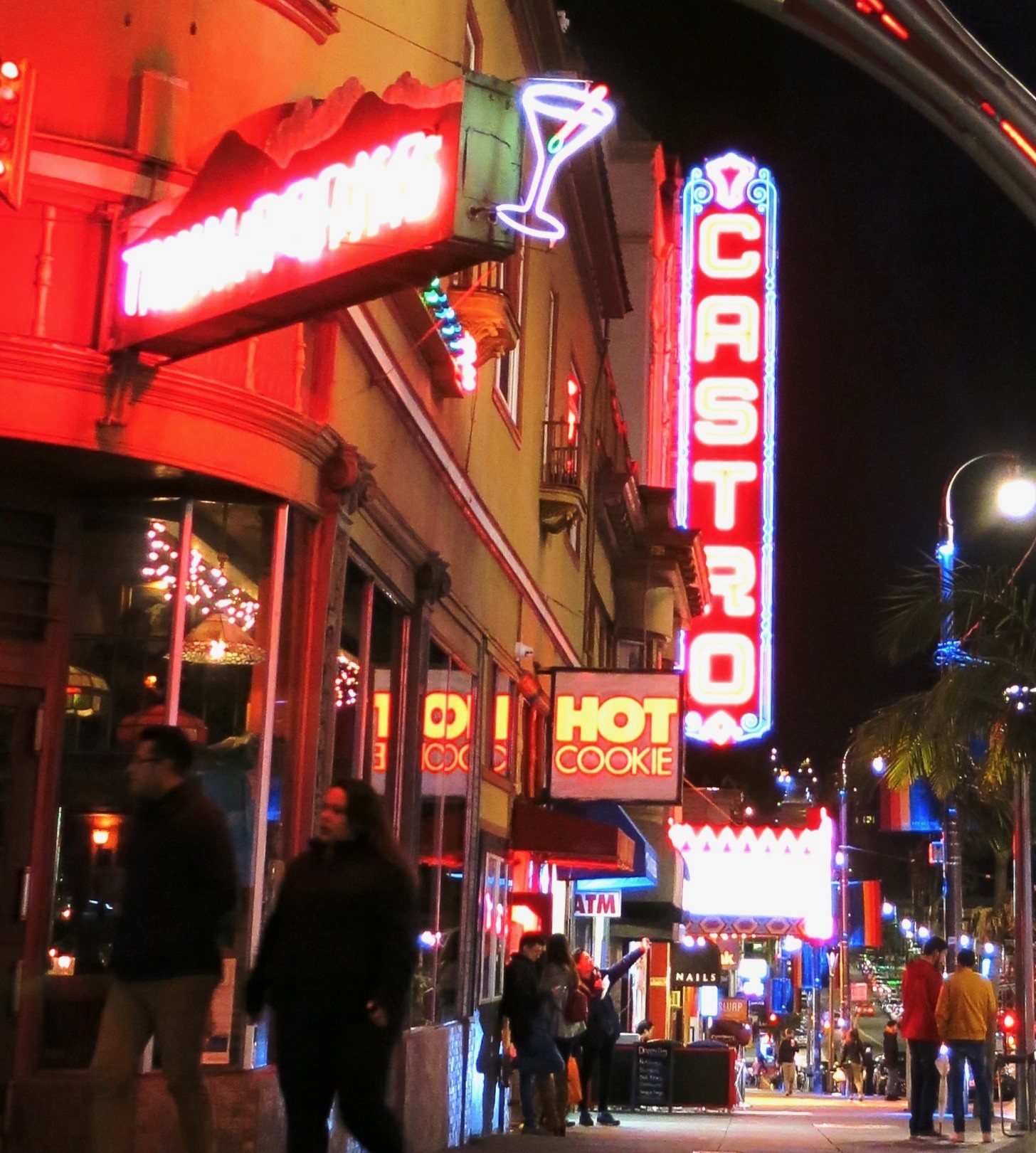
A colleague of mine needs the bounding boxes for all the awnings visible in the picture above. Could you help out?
[512,799,659,891]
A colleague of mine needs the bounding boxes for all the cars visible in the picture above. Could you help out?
[968,1071,1017,1101]
[795,990,811,1049]
[872,1051,907,1097]
[746,1056,807,1091]
[857,954,906,1025]
[854,1000,875,1017]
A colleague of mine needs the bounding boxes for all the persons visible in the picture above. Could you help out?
[87,723,238,1153]
[777,1028,800,1095]
[500,931,586,1137]
[901,936,949,1140]
[245,777,419,1153]
[573,938,652,1125]
[884,1020,901,1100]
[636,1020,654,1040]
[752,1056,762,1087]
[839,1028,866,1102]
[934,949,997,1141]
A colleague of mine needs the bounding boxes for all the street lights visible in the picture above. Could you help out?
[840,736,884,1012]
[838,844,918,947]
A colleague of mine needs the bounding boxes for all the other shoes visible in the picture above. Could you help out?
[521,1124,555,1136]
[910,1130,950,1145]
[597,1112,621,1126]
[500,1053,513,1088]
[951,1131,965,1143]
[579,1113,594,1126]
[982,1132,996,1144]
[566,1120,577,1125]
[887,1095,899,1100]
[558,1121,566,1137]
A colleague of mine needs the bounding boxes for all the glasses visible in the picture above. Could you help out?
[132,755,169,766]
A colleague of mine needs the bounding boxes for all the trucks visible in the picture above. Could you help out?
[850,983,867,1003]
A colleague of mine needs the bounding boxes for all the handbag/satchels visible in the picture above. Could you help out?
[564,1055,583,1104]
[833,1067,846,1082]
[566,963,587,1023]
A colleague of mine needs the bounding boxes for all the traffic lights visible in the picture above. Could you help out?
[768,1012,779,1025]
[1003,1012,1018,1032]
